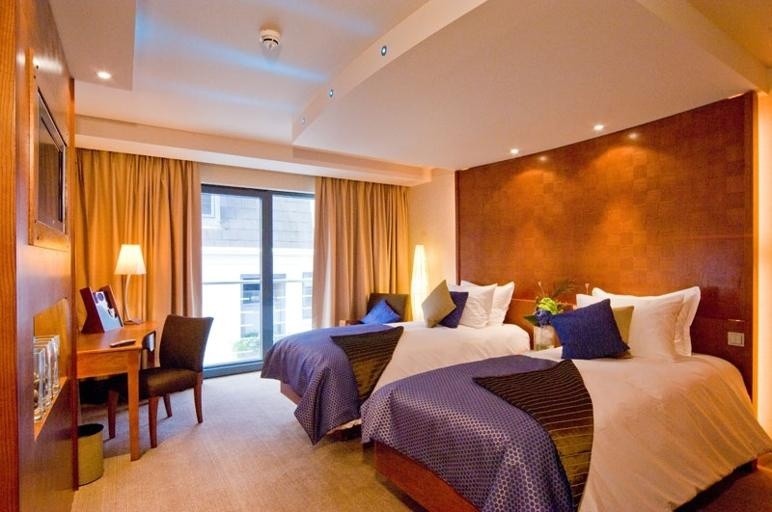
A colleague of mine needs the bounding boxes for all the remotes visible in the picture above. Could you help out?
[109,339,136,347]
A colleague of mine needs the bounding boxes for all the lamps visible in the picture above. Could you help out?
[114,243,149,325]
[260,29,281,52]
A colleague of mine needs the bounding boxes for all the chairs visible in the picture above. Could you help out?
[108,316,214,449]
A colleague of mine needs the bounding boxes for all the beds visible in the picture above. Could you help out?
[261,298,572,449]
[359,318,751,512]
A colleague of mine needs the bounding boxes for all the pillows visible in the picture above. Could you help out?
[592,286,702,358]
[612,305,634,360]
[439,291,468,328]
[359,299,400,325]
[421,280,456,327]
[448,282,497,329]
[460,279,515,327]
[549,299,629,360]
[576,294,683,364]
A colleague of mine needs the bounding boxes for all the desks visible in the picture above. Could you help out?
[76,319,156,462]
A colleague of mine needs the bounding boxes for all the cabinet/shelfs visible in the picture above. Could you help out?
[32,294,71,441]
